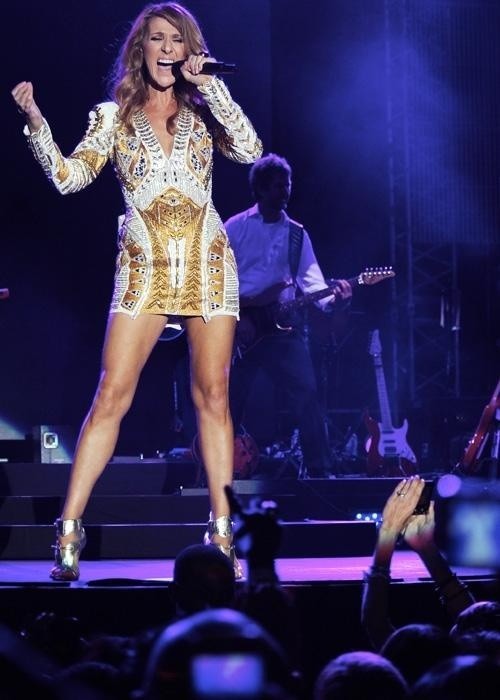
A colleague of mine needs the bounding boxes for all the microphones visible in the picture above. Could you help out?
[171,59,239,76]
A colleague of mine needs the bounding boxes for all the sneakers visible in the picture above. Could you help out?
[306,467,333,479]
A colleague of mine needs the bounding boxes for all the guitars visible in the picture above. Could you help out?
[365,329,417,476]
[465,384,499,469]
[232,267,396,365]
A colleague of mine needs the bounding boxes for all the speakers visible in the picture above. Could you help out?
[33,424,113,467]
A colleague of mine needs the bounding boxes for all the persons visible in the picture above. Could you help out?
[11,0,262,579]
[223,154,353,473]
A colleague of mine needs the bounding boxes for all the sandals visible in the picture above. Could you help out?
[49,518,87,582]
[202,511,245,579]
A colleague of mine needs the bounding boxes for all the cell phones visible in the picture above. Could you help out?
[412,479,434,515]
